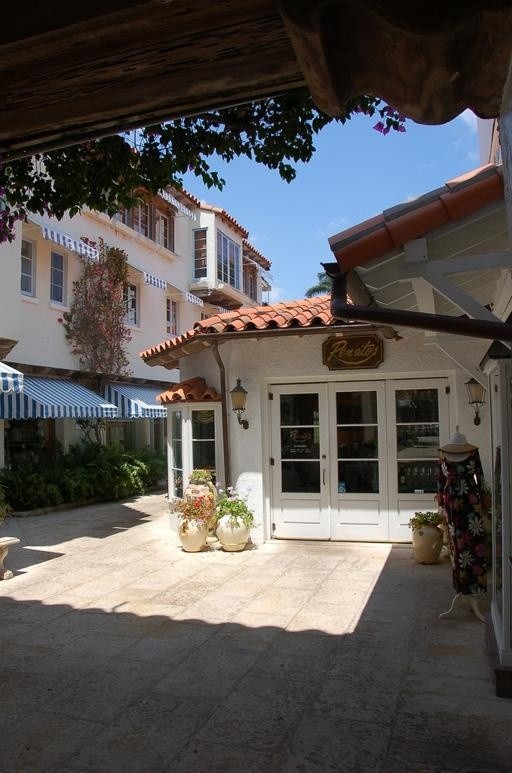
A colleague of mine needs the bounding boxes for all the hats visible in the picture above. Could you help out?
[439,442,480,455]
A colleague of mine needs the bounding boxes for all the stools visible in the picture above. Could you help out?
[0,537,20,580]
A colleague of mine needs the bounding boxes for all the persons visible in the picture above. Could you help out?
[436,434,491,596]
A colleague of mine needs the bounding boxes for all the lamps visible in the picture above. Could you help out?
[229,379,250,430]
[463,368,486,426]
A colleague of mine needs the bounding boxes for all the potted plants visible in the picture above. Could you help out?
[185,470,217,502]
[211,498,253,552]
[170,495,217,552]
[409,511,443,563]
